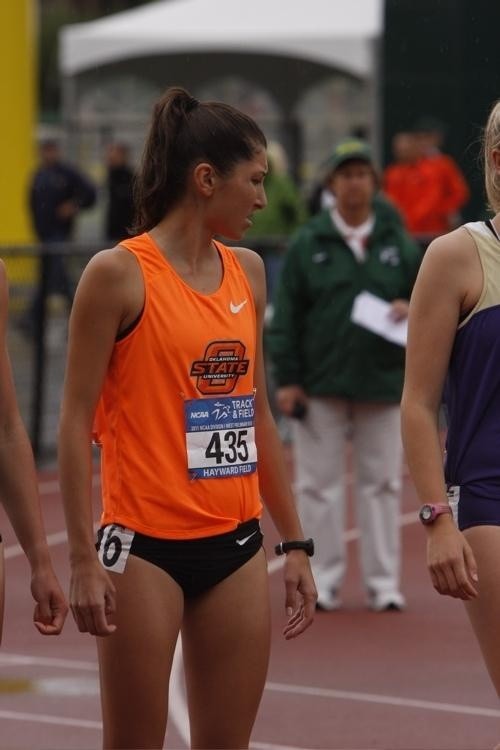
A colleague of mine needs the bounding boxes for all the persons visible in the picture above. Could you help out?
[1,259,71,652]
[398,100,498,704]
[379,125,470,250]
[6,131,99,343]
[65,79,319,748]
[100,136,142,250]
[265,135,421,615]
[401,108,466,228]
[229,150,313,446]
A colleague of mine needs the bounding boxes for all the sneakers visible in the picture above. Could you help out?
[316,584,343,611]
[367,589,406,613]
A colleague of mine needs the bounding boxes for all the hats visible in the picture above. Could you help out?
[326,140,376,177]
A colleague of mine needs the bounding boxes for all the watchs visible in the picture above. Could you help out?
[273,536,315,558]
[416,502,454,525]
